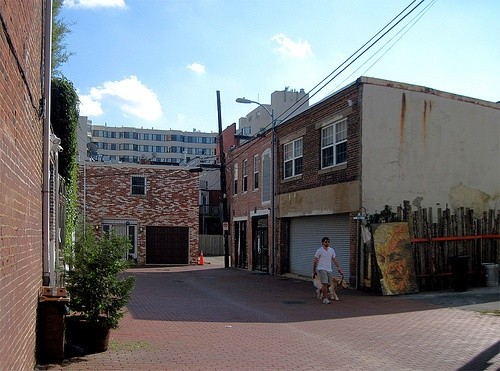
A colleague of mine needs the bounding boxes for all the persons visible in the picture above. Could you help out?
[372,222,420,295]
[312,236,343,304]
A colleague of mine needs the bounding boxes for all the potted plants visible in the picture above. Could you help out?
[65,231,137,354]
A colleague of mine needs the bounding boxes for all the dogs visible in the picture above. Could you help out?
[311,271,349,301]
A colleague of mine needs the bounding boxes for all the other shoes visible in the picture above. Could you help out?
[323,299,331,304]
[316,290,320,300]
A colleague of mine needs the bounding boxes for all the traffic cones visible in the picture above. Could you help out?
[198,249,203,265]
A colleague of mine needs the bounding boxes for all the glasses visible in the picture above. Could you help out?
[324,241,330,243]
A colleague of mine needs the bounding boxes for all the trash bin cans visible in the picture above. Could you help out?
[482,263,498,286]
[447,255,469,292]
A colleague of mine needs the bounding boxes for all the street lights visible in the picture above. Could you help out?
[236,97,276,276]
